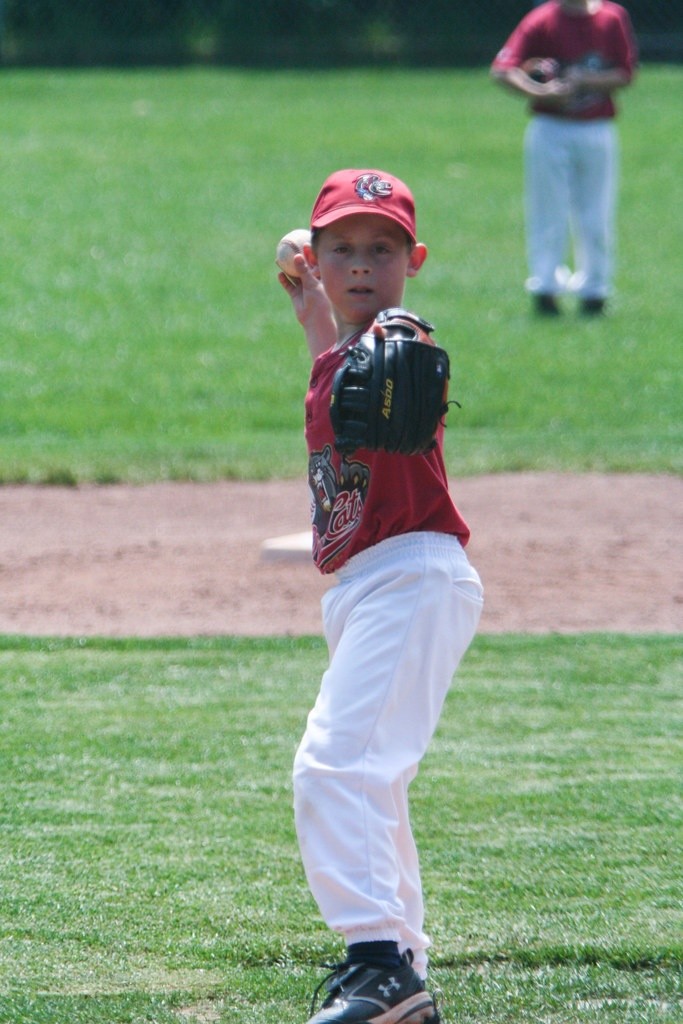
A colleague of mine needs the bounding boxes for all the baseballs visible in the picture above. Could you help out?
[273,227,319,278]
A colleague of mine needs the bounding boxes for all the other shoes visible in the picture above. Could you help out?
[535,294,557,317]
[579,298,604,316]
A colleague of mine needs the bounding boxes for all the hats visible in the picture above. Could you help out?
[311,169,417,245]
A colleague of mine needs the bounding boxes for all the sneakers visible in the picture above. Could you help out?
[305,947,443,1024]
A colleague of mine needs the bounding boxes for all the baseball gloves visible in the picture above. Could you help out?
[327,305,451,457]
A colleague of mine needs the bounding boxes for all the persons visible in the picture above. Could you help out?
[277,167,485,1023]
[488,0,637,314]
